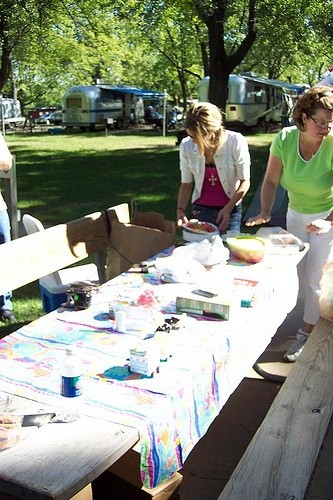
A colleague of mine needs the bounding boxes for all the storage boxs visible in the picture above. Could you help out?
[177,293,235,320]
[106,210,177,281]
[22,214,100,312]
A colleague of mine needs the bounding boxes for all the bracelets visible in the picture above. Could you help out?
[177,206,186,212]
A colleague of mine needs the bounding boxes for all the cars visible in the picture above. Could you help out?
[37,110,62,125]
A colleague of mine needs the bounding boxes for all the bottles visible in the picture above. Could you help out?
[57,347,84,423]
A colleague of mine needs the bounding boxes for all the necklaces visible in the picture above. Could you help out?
[203,152,218,186]
[300,131,323,157]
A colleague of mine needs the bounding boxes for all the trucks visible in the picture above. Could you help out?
[0,97,26,129]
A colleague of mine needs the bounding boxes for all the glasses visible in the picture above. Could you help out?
[308,115,332,128]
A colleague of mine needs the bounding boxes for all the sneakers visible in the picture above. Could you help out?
[283,329,310,362]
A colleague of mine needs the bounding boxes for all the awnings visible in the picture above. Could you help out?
[246,77,308,91]
[101,86,164,97]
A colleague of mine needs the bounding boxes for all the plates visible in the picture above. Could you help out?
[182,222,217,234]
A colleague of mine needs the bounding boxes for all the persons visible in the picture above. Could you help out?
[243,83,333,362]
[176,101,251,239]
[0,132,17,324]
[144,104,184,131]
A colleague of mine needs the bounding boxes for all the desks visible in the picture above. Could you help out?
[0,233,310,500]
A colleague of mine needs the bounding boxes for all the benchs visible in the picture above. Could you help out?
[0,202,130,338]
[217,316,333,500]
